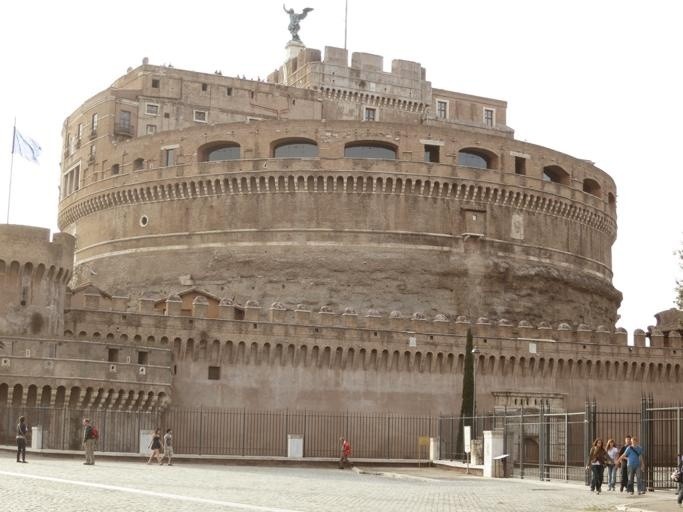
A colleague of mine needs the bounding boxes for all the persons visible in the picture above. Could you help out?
[146,426,163,465]
[603,438,620,491]
[616,435,633,492]
[337,437,353,468]
[158,428,175,465]
[81,418,94,464]
[614,435,645,495]
[15,415,28,463]
[584,438,615,494]
